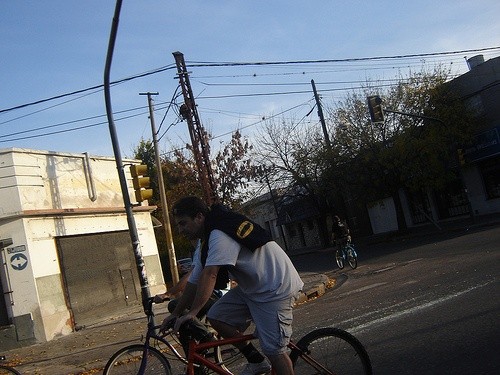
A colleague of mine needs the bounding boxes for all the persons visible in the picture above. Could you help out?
[151,236,232,375]
[332,216,352,261]
[158,195,304,375]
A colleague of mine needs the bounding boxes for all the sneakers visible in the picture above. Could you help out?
[239,360,270,375]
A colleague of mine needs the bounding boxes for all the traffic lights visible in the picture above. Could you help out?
[366,93,387,123]
[128,163,154,202]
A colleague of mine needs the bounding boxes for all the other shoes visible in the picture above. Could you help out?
[199,333,218,354]
[186,365,206,375]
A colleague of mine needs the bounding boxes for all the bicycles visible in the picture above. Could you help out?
[330,237,358,270]
[102,296,290,375]
[156,319,374,375]
